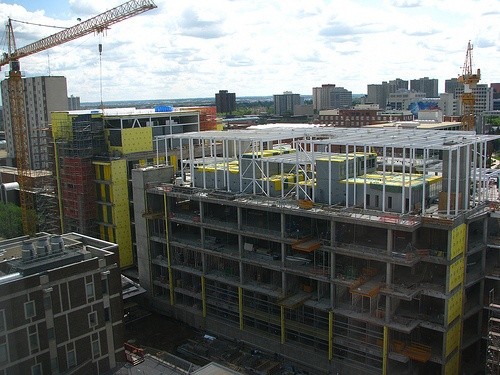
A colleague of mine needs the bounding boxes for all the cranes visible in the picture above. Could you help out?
[1,0,158,239]
[456,40,481,132]
